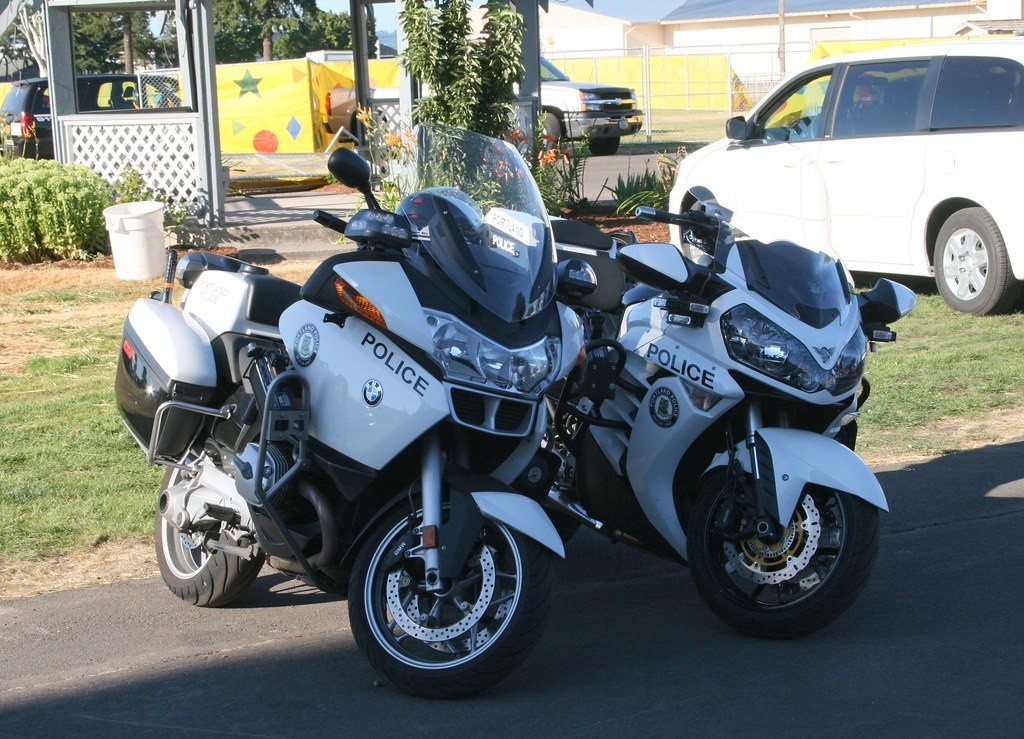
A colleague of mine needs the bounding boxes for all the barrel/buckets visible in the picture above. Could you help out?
[103,200,167,281]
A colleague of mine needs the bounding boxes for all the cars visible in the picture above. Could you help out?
[666,39,1024,316]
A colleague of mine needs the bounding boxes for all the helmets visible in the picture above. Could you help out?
[397,186,493,246]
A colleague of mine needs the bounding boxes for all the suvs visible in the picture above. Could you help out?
[0,74,182,164]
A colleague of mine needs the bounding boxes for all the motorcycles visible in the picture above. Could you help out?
[109,126,629,702]
[542,203,922,645]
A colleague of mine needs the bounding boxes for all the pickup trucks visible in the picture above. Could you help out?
[323,46,646,157]
